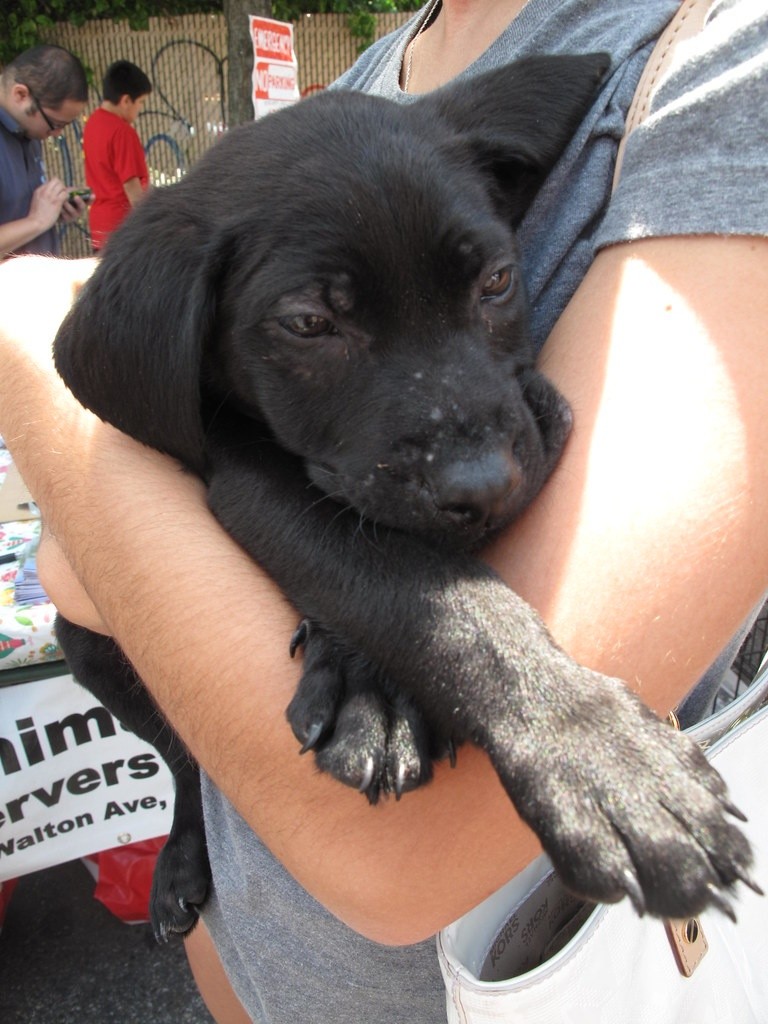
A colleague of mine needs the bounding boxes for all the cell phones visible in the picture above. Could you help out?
[65,188,92,204]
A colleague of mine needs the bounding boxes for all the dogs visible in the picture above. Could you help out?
[53,51,764,925]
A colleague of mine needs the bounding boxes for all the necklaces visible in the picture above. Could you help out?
[405,0,530,94]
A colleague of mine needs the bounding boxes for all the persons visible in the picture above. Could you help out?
[0,44,96,261]
[0,0,768,1024]
[83,59,151,257]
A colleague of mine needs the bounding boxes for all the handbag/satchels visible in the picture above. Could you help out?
[436,650,767,1023]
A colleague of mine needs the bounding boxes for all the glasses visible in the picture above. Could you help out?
[22,86,70,132]
